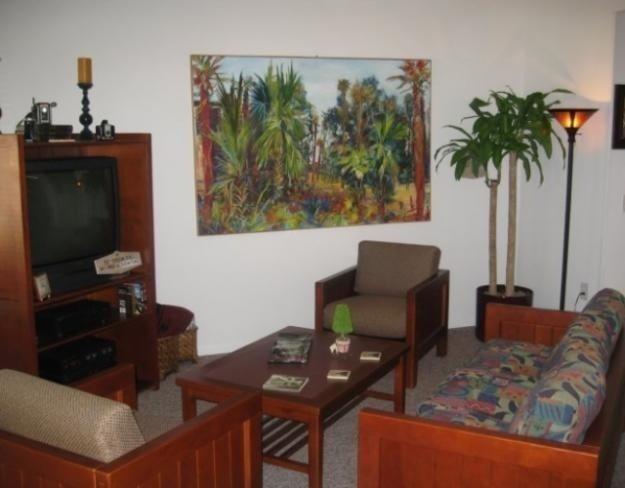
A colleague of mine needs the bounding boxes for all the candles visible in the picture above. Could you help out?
[76,55,93,85]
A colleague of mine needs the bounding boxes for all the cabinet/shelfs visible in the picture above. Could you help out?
[0,130,158,411]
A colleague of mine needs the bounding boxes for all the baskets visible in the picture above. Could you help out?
[158,325,197,371]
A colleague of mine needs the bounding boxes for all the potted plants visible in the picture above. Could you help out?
[432,81,578,340]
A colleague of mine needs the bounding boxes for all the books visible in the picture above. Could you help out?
[360,350,383,360]
[268,332,312,363]
[326,368,351,381]
[262,374,308,392]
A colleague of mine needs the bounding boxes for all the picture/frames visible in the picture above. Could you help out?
[610,83,625,150]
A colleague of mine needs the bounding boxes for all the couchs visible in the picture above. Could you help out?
[352,281,624,487]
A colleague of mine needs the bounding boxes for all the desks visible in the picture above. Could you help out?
[172,320,415,488]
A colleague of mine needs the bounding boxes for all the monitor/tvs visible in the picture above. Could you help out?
[27,156,120,296]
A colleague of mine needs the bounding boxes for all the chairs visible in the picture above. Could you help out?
[313,234,454,391]
[0,364,270,488]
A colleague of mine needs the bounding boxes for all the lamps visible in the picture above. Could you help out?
[545,102,603,314]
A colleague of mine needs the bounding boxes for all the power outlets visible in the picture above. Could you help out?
[578,281,589,301]
